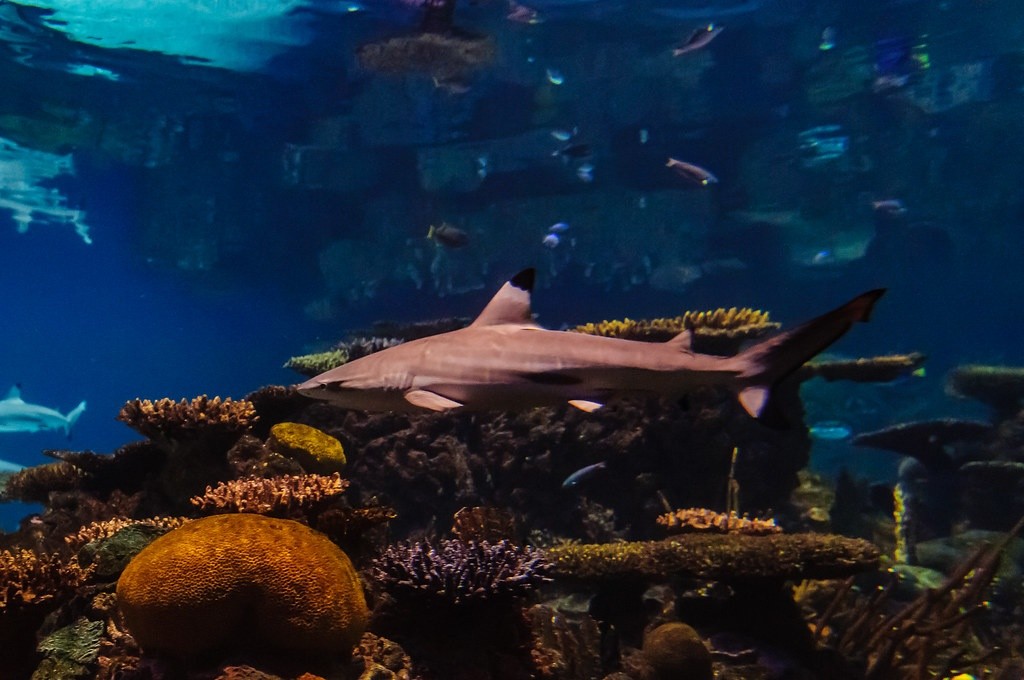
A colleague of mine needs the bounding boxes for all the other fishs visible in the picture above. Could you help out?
[354,11,953,333]
